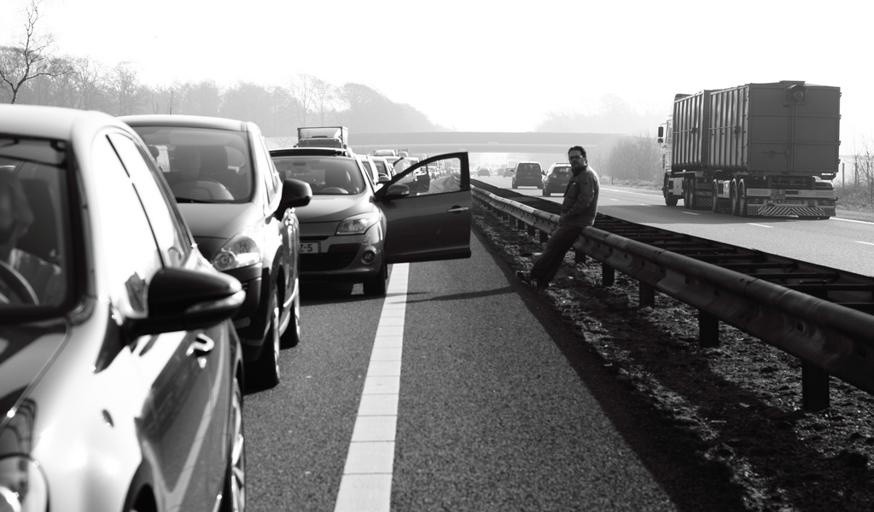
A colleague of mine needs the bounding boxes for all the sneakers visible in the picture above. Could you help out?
[516,271,533,280]
[521,277,542,291]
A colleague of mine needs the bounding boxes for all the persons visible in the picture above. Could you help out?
[514,143,600,291]
[1,165,64,307]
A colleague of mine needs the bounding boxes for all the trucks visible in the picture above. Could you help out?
[655,79,843,223]
[295,123,349,149]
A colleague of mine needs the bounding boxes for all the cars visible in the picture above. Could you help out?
[254,148,474,303]
[111,111,307,390]
[475,164,515,179]
[0,100,249,512]
[538,163,570,196]
[508,160,543,192]
[358,146,461,193]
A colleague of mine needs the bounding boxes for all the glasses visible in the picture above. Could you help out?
[568,155,583,160]
